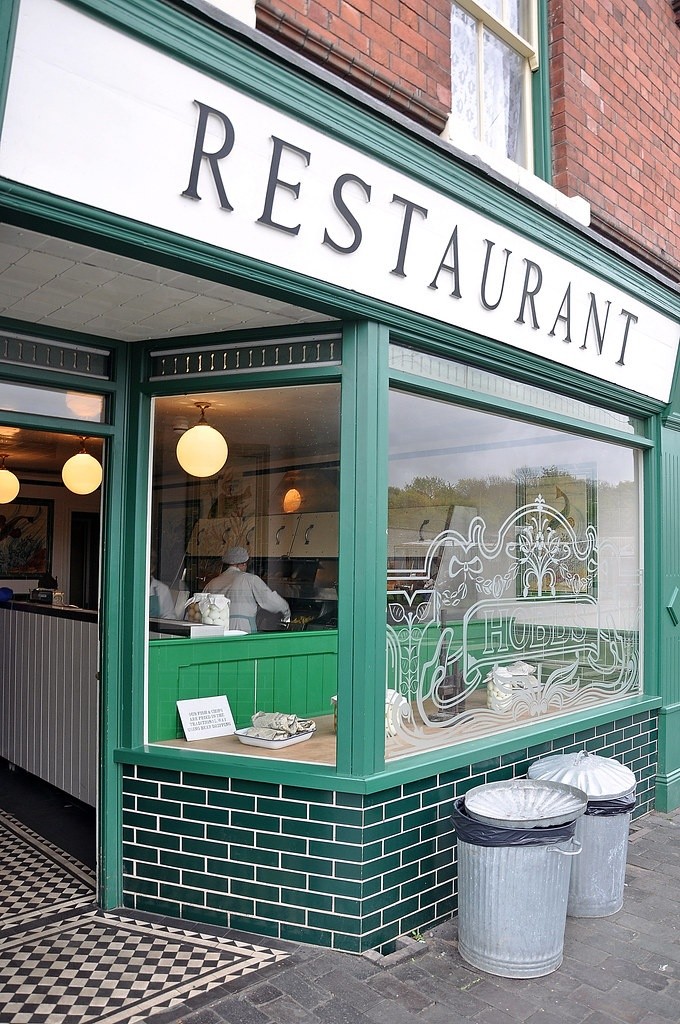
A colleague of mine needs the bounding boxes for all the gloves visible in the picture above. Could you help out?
[282,608,291,623]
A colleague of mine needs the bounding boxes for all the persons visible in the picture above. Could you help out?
[149,563,177,620]
[201,547,290,633]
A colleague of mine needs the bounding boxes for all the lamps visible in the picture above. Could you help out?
[0,454,20,504]
[176,401,228,477]
[61,436,102,494]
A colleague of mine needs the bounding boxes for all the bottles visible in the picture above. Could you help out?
[188,592,230,630]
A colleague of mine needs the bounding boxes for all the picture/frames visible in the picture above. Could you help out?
[157,499,201,581]
[0,497,55,580]
[515,462,597,604]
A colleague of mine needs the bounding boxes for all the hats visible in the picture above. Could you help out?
[221,547,249,565]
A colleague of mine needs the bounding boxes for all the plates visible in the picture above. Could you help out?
[234,727,317,750]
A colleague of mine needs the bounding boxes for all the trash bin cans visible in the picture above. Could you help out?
[526,751,636,918]
[452,779,589,979]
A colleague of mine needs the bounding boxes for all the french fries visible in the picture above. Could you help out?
[290,615,314,623]
